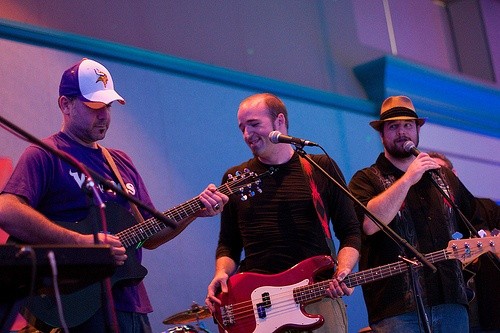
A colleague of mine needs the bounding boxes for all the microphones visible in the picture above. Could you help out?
[403,140,440,175]
[268,130,317,147]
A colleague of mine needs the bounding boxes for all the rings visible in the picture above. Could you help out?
[214,204,219,211]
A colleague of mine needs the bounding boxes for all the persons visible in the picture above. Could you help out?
[427,152,500,333]
[349,96,500,333]
[205,93,363,333]
[0,58,229,333]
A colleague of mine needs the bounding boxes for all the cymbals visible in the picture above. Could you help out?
[163,305,211,324]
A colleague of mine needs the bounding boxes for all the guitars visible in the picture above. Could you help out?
[5,167,262,327]
[211,228,500,333]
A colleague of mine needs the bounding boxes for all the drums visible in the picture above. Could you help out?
[161,325,199,333]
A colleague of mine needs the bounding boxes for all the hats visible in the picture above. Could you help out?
[59,57,126,110]
[369,96,426,132]
